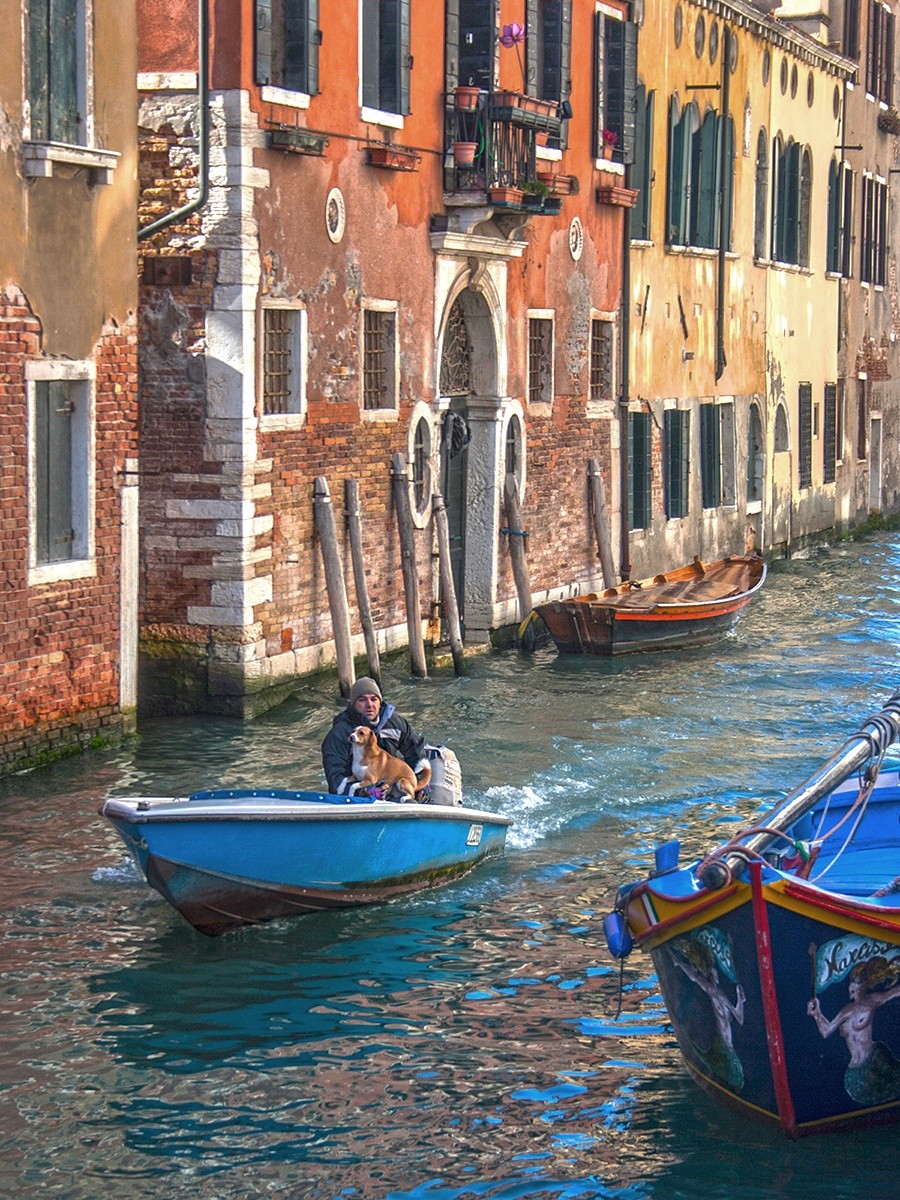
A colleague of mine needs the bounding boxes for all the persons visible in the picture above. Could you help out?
[321,677,431,803]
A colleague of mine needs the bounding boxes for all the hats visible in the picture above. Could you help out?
[351,676,382,705]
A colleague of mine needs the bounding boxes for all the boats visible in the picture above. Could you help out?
[532,555,767,656]
[617,691,900,1139]
[98,744,514,935]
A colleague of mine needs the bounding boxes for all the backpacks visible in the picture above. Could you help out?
[425,744,463,808]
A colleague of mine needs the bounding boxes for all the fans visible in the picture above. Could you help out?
[489,189,523,205]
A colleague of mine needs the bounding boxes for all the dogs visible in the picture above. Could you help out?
[336,726,432,803]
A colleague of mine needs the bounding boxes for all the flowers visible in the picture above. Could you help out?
[602,129,620,146]
[499,20,528,48]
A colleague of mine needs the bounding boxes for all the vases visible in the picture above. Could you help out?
[450,86,480,113]
[520,93,558,117]
[454,139,478,167]
[602,143,615,159]
[538,172,573,196]
[597,186,639,208]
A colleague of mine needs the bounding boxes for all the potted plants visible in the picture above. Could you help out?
[518,180,546,205]
[360,141,419,170]
[271,130,328,153]
[497,91,518,109]
